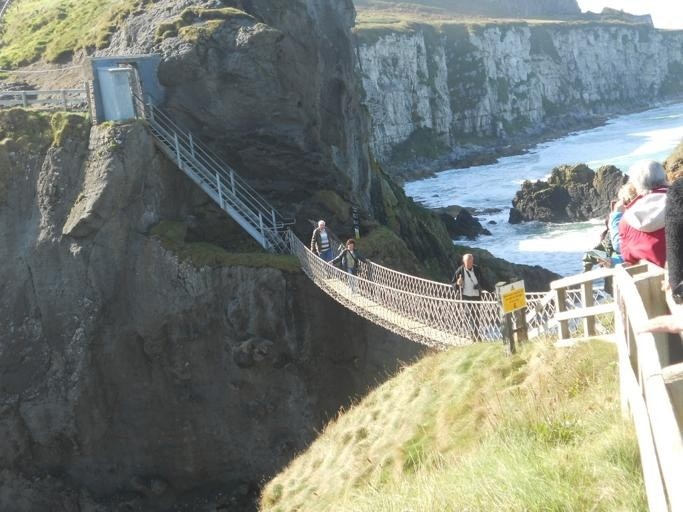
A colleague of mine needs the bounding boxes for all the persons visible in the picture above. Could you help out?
[309,220,344,280]
[581,156,683,369]
[450,253,497,343]
[327,239,370,297]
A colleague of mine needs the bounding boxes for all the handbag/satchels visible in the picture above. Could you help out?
[356,263,361,272]
